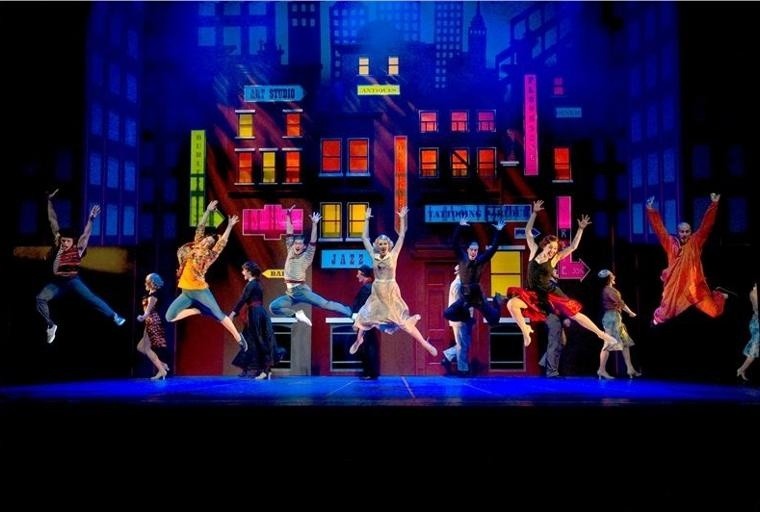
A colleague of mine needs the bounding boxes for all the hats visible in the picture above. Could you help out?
[597,269,612,278]
[454,265,460,274]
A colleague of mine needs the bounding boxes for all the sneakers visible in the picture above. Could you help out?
[46,324,57,344]
[495,293,506,301]
[350,339,365,355]
[716,287,737,300]
[113,313,126,327]
[295,309,312,328]
[540,365,566,379]
[358,372,377,380]
[237,332,250,353]
[425,343,438,358]
[440,357,479,378]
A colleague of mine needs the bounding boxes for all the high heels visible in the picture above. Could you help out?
[736,367,749,380]
[254,369,273,381]
[152,364,170,381]
[523,324,536,347]
[627,368,642,378]
[609,336,617,347]
[597,369,615,380]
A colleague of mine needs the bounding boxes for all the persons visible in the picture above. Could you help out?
[164,197,249,354]
[442,214,510,329]
[135,270,172,384]
[347,205,439,359]
[537,265,567,380]
[439,262,478,379]
[736,280,760,383]
[594,265,643,383]
[350,265,379,381]
[226,260,286,384]
[508,198,618,346]
[643,190,740,330]
[34,187,126,345]
[267,202,359,329]
